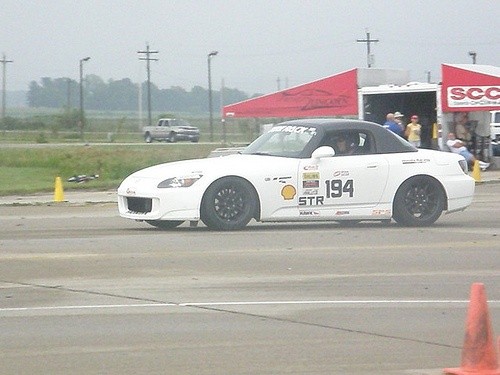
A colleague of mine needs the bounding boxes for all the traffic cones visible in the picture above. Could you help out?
[48,176,69,202]
[471,159,484,183]
[442,284,500,375]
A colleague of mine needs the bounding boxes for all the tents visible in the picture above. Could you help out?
[442,64,500,113]
[222,67,410,143]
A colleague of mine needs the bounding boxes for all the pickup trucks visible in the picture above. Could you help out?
[142,118,200,143]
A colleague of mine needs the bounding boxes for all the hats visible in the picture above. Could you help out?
[394,111,404,118]
[411,115,419,119]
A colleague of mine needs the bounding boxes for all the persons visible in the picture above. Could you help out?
[383,111,405,138]
[447,134,491,172]
[331,131,355,155]
[456,115,473,151]
[404,115,422,148]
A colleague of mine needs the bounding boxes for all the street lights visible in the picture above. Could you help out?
[469,51,478,64]
[207,51,218,140]
[78,56,92,142]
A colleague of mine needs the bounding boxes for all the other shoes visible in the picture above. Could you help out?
[479,161,490,171]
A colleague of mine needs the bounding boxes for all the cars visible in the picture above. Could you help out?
[116,118,476,230]
